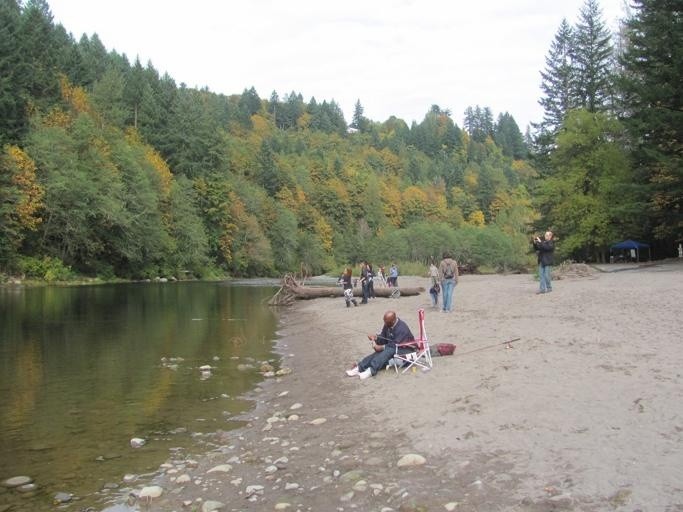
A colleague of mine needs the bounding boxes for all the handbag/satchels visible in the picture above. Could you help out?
[430,285,440,292]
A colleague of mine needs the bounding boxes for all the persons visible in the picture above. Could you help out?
[429,283,440,309]
[532,231,555,294]
[428,263,439,285]
[439,250,459,312]
[336,259,398,307]
[345,311,418,381]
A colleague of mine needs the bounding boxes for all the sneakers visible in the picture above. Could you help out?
[358,366,371,380]
[345,366,359,376]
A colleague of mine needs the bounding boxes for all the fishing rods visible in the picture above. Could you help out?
[435,338,521,357]
[341,325,393,343]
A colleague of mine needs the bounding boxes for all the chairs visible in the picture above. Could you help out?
[392,308,434,376]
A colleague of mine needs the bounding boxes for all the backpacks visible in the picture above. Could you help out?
[443,260,453,279]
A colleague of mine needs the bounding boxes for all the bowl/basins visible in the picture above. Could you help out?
[436,343,456,355]
